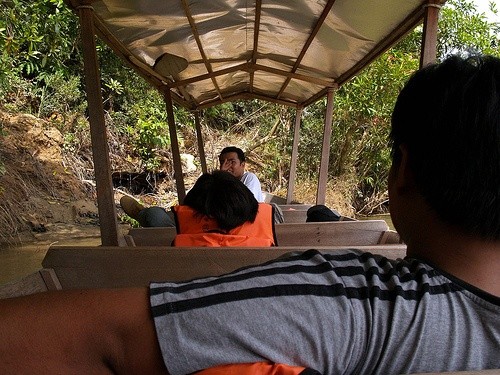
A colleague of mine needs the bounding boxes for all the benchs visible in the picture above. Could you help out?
[0,188,407,299]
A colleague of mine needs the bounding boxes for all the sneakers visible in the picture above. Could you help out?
[120,195,146,222]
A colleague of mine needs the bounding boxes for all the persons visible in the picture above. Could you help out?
[172,169,277,247]
[219,146,264,203]
[120,195,176,227]
[1,56,500,375]
